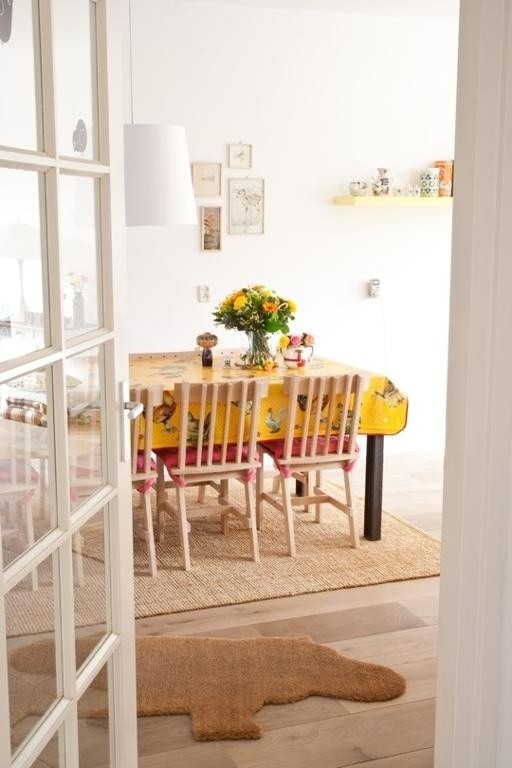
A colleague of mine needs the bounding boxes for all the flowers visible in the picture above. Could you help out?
[212,285,297,364]
[279,333,316,350]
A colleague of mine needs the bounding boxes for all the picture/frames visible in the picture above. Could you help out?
[228,143,252,170]
[201,205,222,251]
[229,177,265,234]
[192,161,222,196]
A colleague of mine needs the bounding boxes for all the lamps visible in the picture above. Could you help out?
[122,1,184,227]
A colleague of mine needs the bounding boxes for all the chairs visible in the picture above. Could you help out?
[257,374,366,557]
[153,376,270,571]
[0,383,157,592]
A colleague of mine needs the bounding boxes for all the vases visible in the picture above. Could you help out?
[281,345,313,369]
[240,332,276,366]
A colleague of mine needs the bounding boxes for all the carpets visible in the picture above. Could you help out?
[1,482,442,637]
[7,637,406,746]
